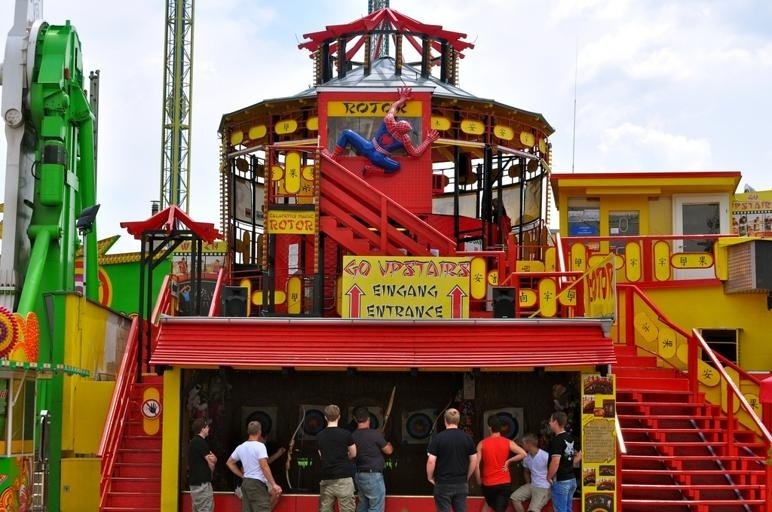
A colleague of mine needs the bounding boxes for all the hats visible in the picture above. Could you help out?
[192,418,213,432]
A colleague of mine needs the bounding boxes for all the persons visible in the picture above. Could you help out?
[212,258,220,273]
[347,406,393,512]
[226,421,282,512]
[492,197,511,244]
[321,86,440,180]
[177,257,188,273]
[509,432,552,512]
[187,419,218,512]
[315,405,357,512]
[474,415,528,512]
[426,408,478,512]
[547,411,582,512]
[180,285,191,315]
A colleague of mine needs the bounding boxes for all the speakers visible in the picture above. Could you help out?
[493,288,515,318]
[223,286,248,317]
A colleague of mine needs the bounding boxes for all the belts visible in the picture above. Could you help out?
[356,469,381,475]
